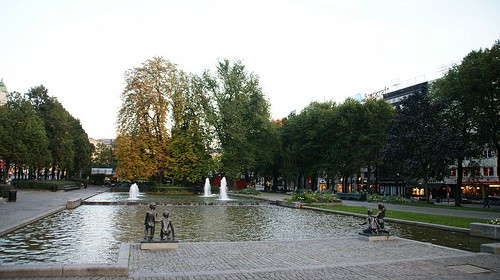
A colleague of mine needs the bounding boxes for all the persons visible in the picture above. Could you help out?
[1,172,117,191]
[160,212,176,242]
[359,209,381,235]
[372,203,387,233]
[143,202,162,242]
[248,177,500,209]
